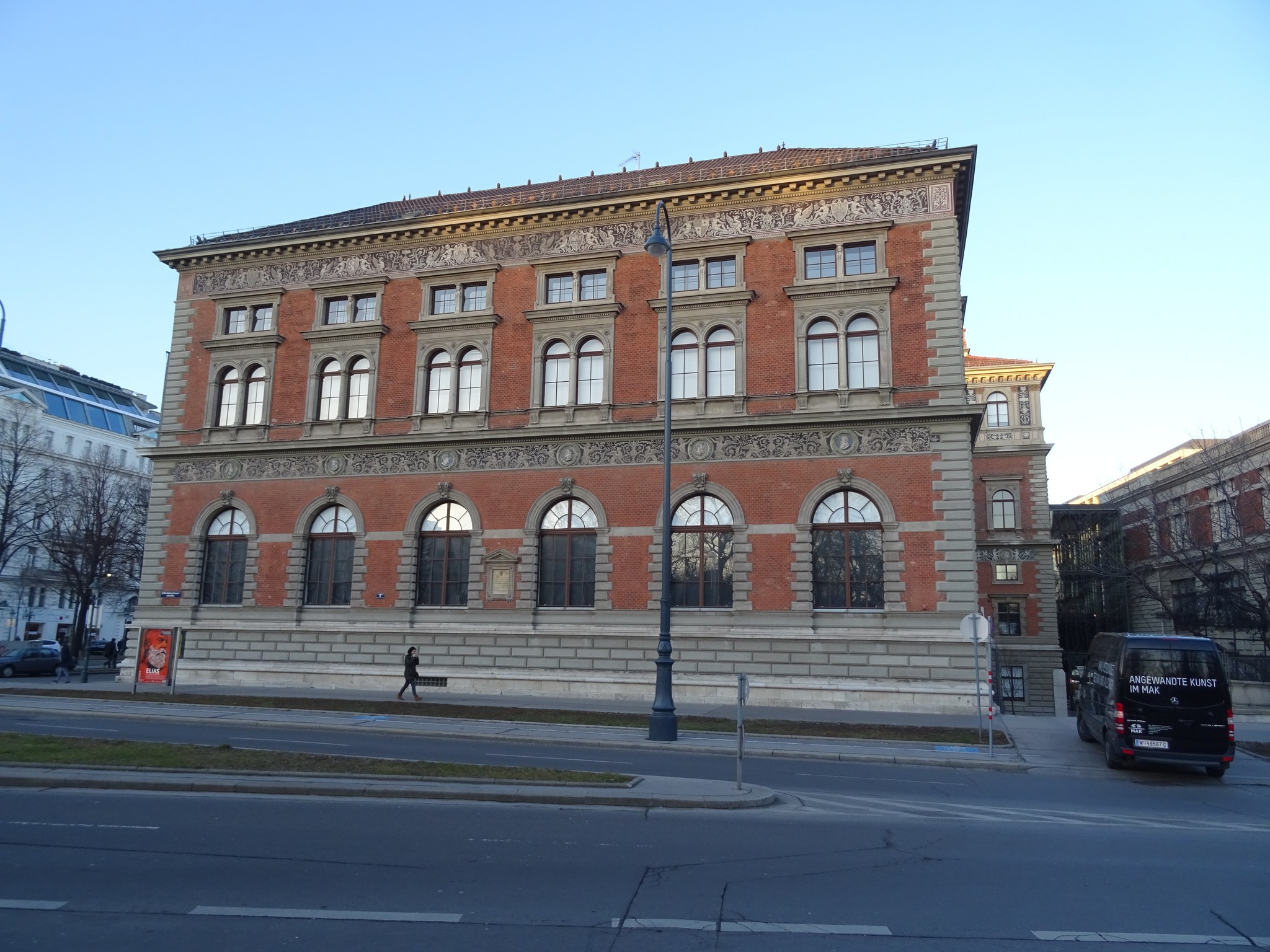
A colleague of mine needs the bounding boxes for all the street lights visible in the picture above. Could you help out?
[80,576,103,683]
[642,200,679,742]
[123,609,130,640]
[7,609,16,641]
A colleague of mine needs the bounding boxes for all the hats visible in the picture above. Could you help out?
[62,643,67,647]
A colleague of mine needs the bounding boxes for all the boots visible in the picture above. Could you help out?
[397,692,404,700]
[414,695,422,701]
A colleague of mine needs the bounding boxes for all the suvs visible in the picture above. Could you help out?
[0,639,62,678]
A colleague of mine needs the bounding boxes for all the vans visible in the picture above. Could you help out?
[1070,633,1235,781]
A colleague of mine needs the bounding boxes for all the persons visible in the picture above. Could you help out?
[103,638,116,668]
[16,636,20,641]
[117,639,126,656]
[397,647,423,701]
[57,637,63,644]
[51,641,73,683]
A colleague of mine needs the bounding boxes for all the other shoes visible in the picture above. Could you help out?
[64,681,70,684]
[52,680,57,683]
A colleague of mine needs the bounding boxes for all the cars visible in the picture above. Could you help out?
[84,639,110,656]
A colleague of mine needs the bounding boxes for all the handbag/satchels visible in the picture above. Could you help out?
[407,670,415,680]
